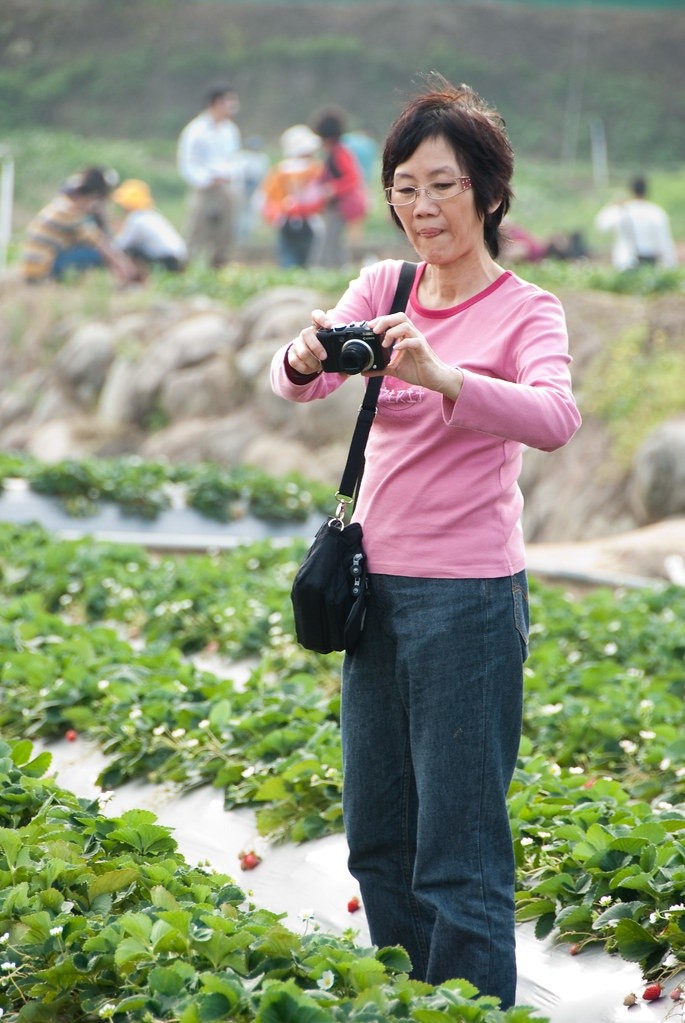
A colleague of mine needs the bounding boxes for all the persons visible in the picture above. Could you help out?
[499,219,601,265]
[14,165,121,290]
[596,173,677,275]
[112,176,190,286]
[267,64,584,1014]
[239,103,383,269]
[175,82,246,277]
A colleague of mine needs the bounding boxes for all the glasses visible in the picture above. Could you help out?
[384,176,472,206]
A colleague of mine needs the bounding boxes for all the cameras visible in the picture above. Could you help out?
[316,320,390,376]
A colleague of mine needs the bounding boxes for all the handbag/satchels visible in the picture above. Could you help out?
[290,517,370,655]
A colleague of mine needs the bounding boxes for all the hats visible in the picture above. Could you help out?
[109,180,154,211]
[279,126,321,157]
